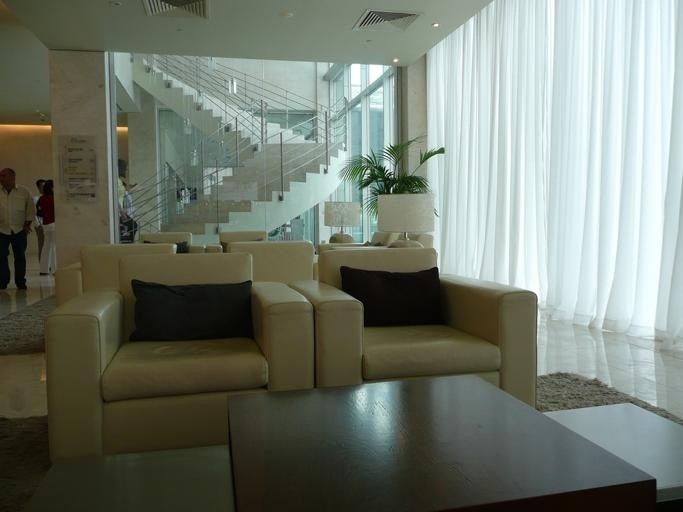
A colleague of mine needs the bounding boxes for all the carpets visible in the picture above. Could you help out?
[0,413,50,512]
[0,295,56,354]
[536,370,682,428]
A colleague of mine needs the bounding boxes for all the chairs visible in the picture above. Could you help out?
[43,247,315,456]
[135,231,206,253]
[291,245,538,410]
[206,230,268,253]
[226,241,314,284]
[55,243,177,305]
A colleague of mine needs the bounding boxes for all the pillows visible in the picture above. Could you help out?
[340,265,444,326]
[144,240,187,253]
[130,277,252,341]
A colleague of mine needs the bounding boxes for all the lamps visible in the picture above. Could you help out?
[324,201,362,243]
[378,192,434,248]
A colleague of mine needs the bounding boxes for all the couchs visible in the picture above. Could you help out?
[316,230,400,252]
[331,233,434,249]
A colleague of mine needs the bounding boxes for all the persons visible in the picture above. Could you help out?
[0,168,37,289]
[33,178,44,262]
[36,179,55,275]
[118,159,138,243]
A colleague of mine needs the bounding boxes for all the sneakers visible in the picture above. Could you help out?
[17,284,27,290]
[1,284,8,289]
